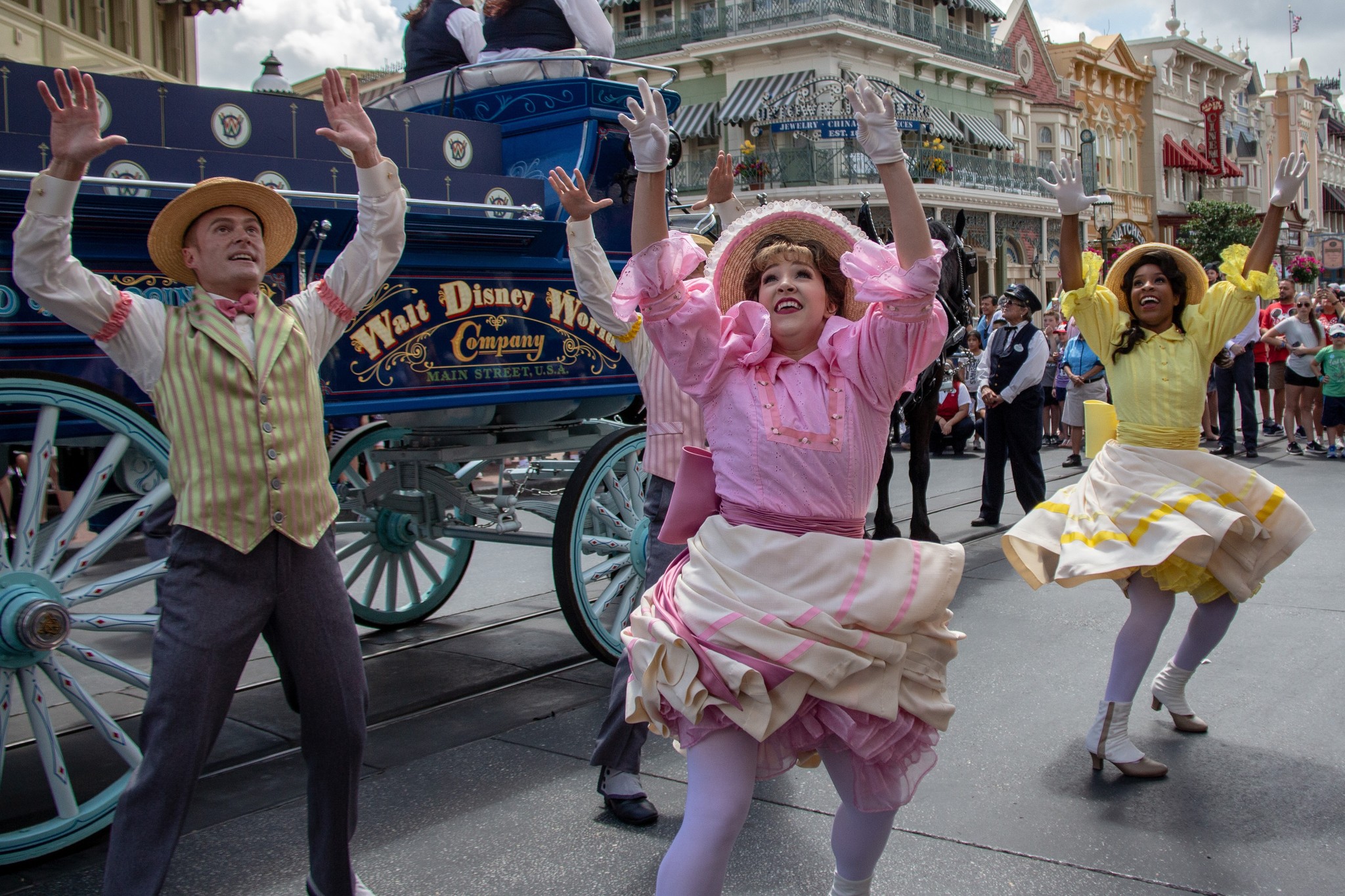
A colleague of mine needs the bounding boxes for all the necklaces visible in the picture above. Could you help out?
[1297,314,1310,322]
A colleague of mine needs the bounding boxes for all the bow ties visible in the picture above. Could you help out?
[213,293,258,323]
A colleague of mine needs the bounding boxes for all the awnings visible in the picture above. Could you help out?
[1163,135,1244,178]
[1322,184,1345,214]
[845,71,1016,151]
[666,101,720,141]
[718,69,816,127]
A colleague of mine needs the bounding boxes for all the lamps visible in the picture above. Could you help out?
[1030,252,1042,282]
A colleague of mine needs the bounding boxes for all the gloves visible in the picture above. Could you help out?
[845,74,905,165]
[1037,157,1099,216]
[1269,152,1311,207]
[618,77,670,173]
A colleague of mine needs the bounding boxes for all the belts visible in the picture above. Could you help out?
[1083,374,1105,383]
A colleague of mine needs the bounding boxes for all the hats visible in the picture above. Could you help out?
[1105,242,1209,320]
[703,199,869,347]
[1006,283,1017,291]
[1052,321,1068,334]
[1327,283,1345,292]
[1204,263,1218,270]
[1329,323,1345,336]
[145,175,299,287]
[1003,284,1042,314]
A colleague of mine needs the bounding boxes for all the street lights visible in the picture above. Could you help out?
[1277,216,1291,279]
[1090,185,1115,285]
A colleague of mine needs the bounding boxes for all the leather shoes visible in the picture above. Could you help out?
[604,794,659,828]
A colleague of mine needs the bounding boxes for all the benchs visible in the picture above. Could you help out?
[375,48,585,112]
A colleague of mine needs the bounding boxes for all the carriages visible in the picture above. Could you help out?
[0,54,984,886]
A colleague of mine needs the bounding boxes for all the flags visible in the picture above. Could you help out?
[1291,12,1301,33]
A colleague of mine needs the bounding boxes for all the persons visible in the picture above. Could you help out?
[404,0,616,84]
[610,74,964,896]
[547,147,749,826]
[890,261,1345,467]
[1002,151,1318,778]
[12,67,407,896]
[0,415,587,598]
[968,285,1050,526]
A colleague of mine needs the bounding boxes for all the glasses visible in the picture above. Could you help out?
[1331,333,1345,338]
[1004,299,1025,308]
[1297,303,1310,308]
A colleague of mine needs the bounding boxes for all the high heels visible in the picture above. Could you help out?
[1151,655,1208,732]
[1085,699,1168,778]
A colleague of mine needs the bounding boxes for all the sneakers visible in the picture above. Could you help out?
[1262,417,1345,459]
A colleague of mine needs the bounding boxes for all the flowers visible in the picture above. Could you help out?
[914,138,954,177]
[1081,243,1135,275]
[1058,269,1061,277]
[732,139,772,183]
[1273,260,1283,279]
[1287,256,1325,285]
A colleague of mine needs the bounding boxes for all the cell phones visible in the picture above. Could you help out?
[1291,341,1306,358]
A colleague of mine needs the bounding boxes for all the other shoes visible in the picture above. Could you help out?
[1062,454,1082,467]
[306,868,374,896]
[1210,445,1235,458]
[1041,429,1086,447]
[1200,425,1220,442]
[1246,448,1258,458]
[971,517,999,526]
[928,440,981,458]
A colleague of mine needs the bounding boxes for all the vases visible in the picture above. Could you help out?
[749,182,765,190]
[923,177,936,184]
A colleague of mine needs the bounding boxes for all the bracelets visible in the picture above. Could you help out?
[1332,300,1340,306]
[1052,387,1056,389]
[1281,341,1285,347]
[1285,342,1287,348]
[1316,375,1324,382]
